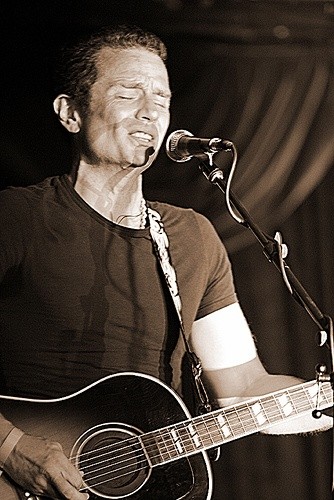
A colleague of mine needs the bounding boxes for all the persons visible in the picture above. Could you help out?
[0,25,334,500]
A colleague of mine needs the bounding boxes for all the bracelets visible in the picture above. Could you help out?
[0,427,25,466]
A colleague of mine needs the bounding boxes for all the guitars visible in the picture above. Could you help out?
[0,364,334,500]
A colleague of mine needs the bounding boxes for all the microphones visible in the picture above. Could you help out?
[166,130,234,162]
